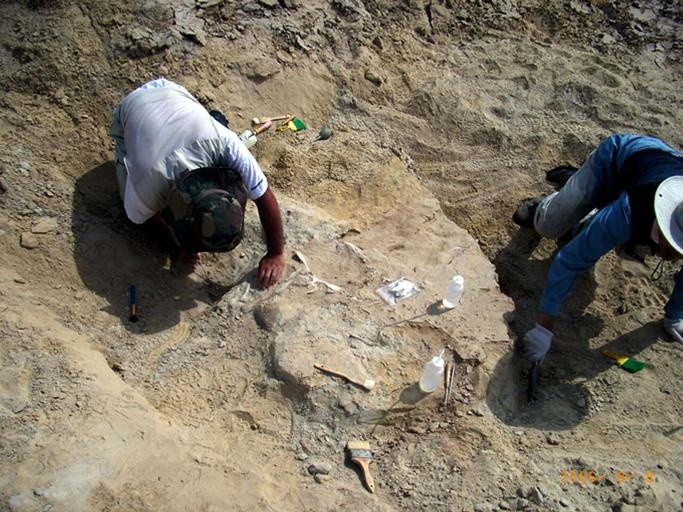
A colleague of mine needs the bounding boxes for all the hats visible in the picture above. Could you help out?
[652,174,682,257]
[160,167,247,253]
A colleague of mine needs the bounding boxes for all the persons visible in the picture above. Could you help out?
[101,78,288,292]
[511,131,683,369]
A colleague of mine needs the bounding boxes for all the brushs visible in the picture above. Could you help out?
[347,441,375,492]
[129,285,139,322]
[314,363,375,390]
[604,350,646,373]
[253,115,290,124]
[280,118,305,132]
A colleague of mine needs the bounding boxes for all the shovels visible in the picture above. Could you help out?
[239,121,272,149]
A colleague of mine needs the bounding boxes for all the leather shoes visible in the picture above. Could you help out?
[546,166,578,184]
[512,197,544,226]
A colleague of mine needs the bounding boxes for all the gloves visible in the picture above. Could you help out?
[662,317,682,345]
[521,321,555,369]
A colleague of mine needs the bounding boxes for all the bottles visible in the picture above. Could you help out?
[442,275,464,308]
[417,356,444,396]
